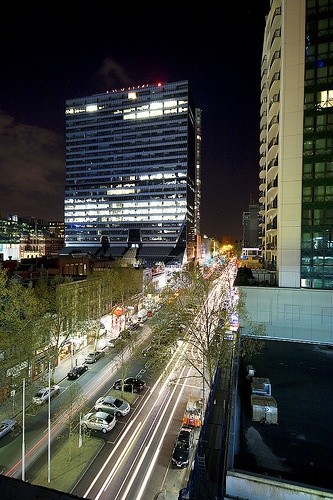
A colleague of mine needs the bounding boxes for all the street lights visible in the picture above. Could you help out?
[180,323,205,421]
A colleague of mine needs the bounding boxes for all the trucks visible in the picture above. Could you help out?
[183,395,206,427]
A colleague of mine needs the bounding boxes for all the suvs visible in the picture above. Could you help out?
[137,315,147,323]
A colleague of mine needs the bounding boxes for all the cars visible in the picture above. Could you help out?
[147,302,166,317]
[80,412,116,434]
[94,396,131,417]
[214,286,239,342]
[0,419,17,439]
[170,425,194,468]
[106,338,118,347]
[129,323,139,330]
[85,350,105,363]
[32,384,60,405]
[119,330,130,339]
[112,377,147,394]
[67,364,88,378]
